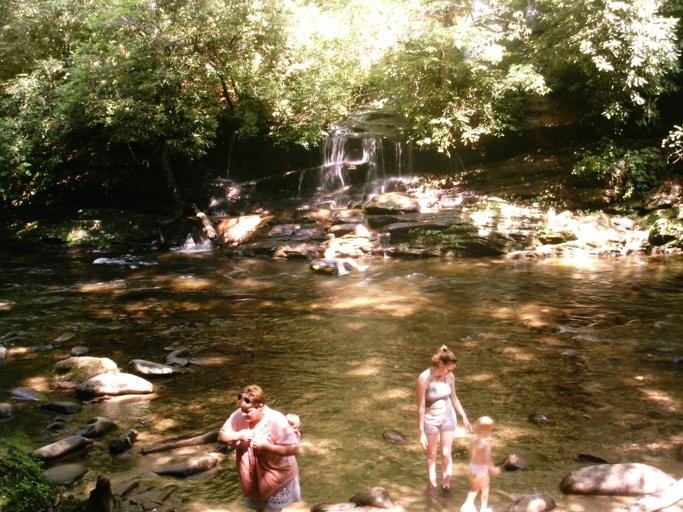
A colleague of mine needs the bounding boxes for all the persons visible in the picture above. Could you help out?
[417,344,473,490]
[461,416,501,512]
[217,385,301,512]
[286,414,301,441]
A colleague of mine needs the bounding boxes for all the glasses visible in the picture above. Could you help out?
[236,393,254,405]
